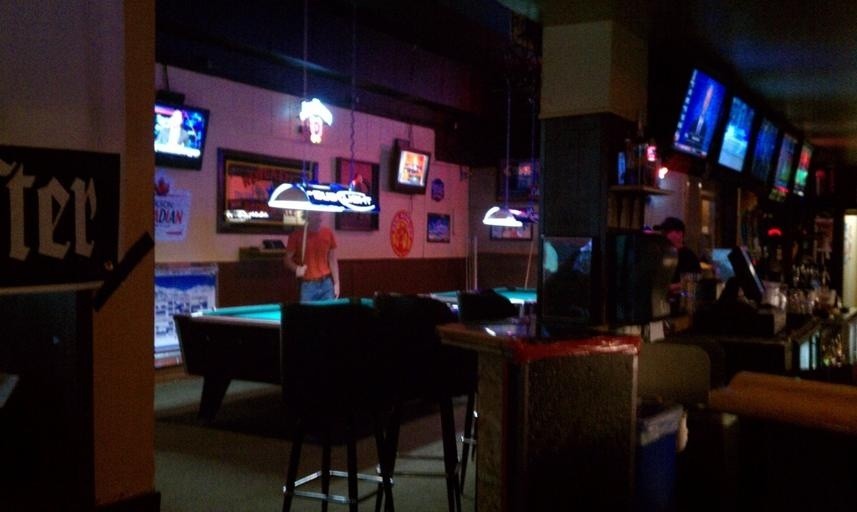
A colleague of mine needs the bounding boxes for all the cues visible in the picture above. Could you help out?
[519,241,534,320]
[301,211,307,266]
[465,233,478,292]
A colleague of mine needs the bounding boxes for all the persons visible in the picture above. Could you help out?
[283,212,341,302]
[660,217,700,274]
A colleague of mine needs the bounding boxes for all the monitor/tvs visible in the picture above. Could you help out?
[153,101,210,170]
[727,246,766,302]
[392,146,431,195]
[648,59,817,207]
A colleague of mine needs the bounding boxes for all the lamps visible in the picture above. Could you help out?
[266,0,380,214]
[481,13,540,228]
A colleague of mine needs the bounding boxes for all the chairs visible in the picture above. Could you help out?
[374,295,459,512]
[459,289,516,493]
[280,300,396,512]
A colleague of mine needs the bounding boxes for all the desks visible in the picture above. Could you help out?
[173,284,538,418]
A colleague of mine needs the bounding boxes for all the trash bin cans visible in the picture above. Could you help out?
[635,396,685,512]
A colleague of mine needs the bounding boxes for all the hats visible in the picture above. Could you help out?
[653,217,685,230]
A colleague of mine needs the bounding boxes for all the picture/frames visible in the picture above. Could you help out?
[337,157,378,231]
[427,213,450,242]
[216,147,320,236]
